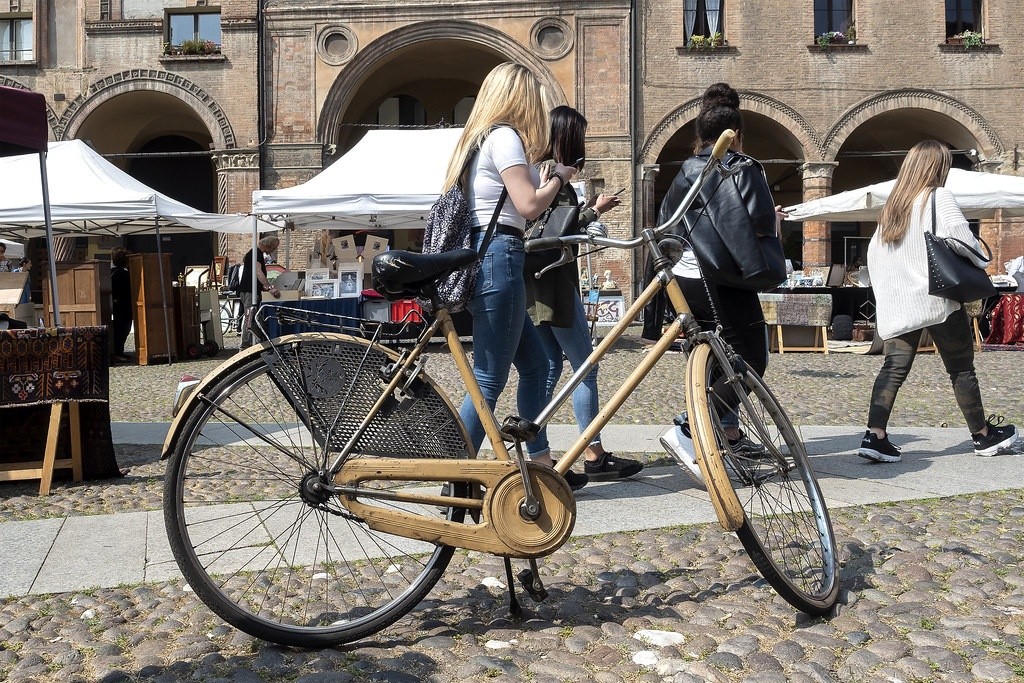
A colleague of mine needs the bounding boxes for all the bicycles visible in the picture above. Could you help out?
[158,125,842,651]
[198,290,246,338]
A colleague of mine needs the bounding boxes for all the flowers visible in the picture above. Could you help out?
[820,31,843,49]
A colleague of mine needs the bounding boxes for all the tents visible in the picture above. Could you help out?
[0,139,287,366]
[0,86,60,325]
[247,129,465,345]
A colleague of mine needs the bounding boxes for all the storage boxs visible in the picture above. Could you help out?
[363,299,392,323]
[261,288,305,301]
[392,300,423,323]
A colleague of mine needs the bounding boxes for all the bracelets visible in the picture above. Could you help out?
[549,172,564,191]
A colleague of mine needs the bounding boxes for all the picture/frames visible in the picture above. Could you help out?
[182,265,210,288]
[305,279,339,298]
[210,256,227,282]
[338,264,362,297]
[306,268,331,279]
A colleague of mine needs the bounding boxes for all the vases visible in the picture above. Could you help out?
[832,37,841,44]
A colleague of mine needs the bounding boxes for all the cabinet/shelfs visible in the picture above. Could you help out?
[126,252,174,364]
[42,260,112,328]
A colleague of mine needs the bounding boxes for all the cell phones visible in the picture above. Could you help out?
[784,208,797,214]
[571,157,585,167]
[613,188,626,196]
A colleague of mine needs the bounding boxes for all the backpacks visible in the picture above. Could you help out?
[422,123,526,314]
[228,264,243,291]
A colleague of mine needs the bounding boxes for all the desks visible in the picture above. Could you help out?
[260,296,362,342]
[0,322,111,499]
[984,292,1024,346]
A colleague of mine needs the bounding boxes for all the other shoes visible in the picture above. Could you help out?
[552,460,589,491]
[111,354,133,363]
[439,484,485,514]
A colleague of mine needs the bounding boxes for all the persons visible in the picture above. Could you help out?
[436,60,591,516]
[110,251,132,364]
[641,80,789,489]
[858,137,1019,462]
[16,257,32,305]
[0,242,15,272]
[236,236,281,352]
[520,105,643,481]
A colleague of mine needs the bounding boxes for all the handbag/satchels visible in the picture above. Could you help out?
[924,188,997,303]
[525,202,584,236]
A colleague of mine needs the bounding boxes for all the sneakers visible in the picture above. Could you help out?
[674,411,692,439]
[719,430,765,456]
[660,424,707,485]
[972,414,1020,457]
[584,453,644,482]
[858,431,902,462]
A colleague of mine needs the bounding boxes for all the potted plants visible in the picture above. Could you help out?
[180,38,216,55]
[948,31,983,49]
[689,31,723,53]
[846,26,856,44]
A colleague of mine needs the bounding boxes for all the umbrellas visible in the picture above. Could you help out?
[781,165,1024,355]
